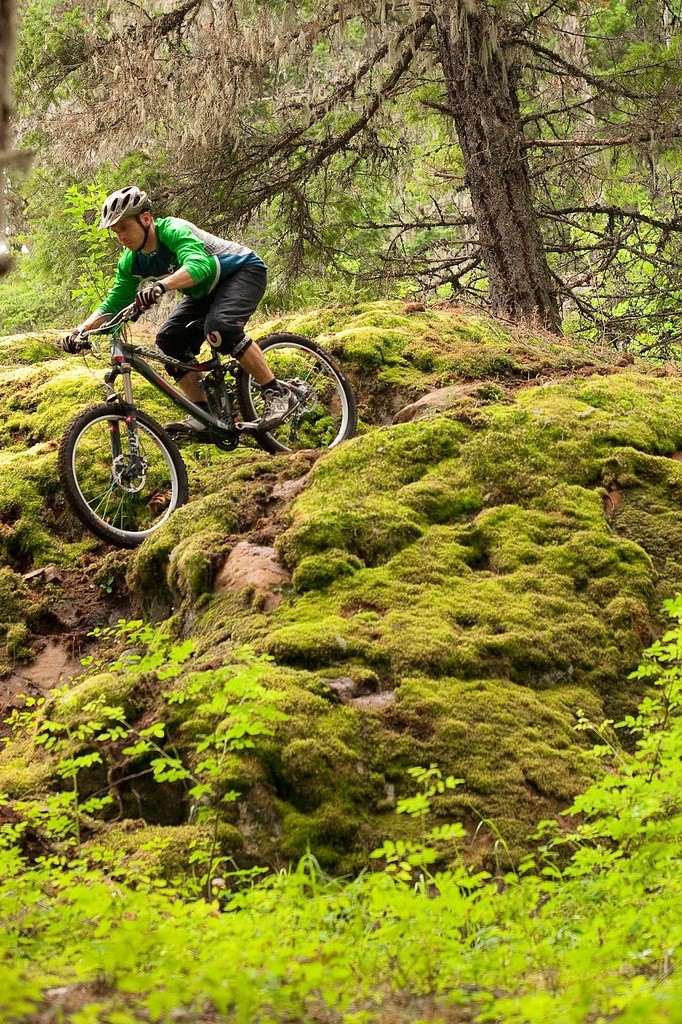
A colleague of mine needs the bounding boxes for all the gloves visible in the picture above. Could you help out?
[61,328,86,353]
[136,280,167,308]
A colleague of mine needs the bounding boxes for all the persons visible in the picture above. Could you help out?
[60,184,299,431]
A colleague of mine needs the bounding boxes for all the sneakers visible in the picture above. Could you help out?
[163,414,207,432]
[258,392,288,430]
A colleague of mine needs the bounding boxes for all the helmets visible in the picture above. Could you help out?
[97,186,154,231]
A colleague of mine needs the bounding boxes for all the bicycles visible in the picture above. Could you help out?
[56,284,357,552]
